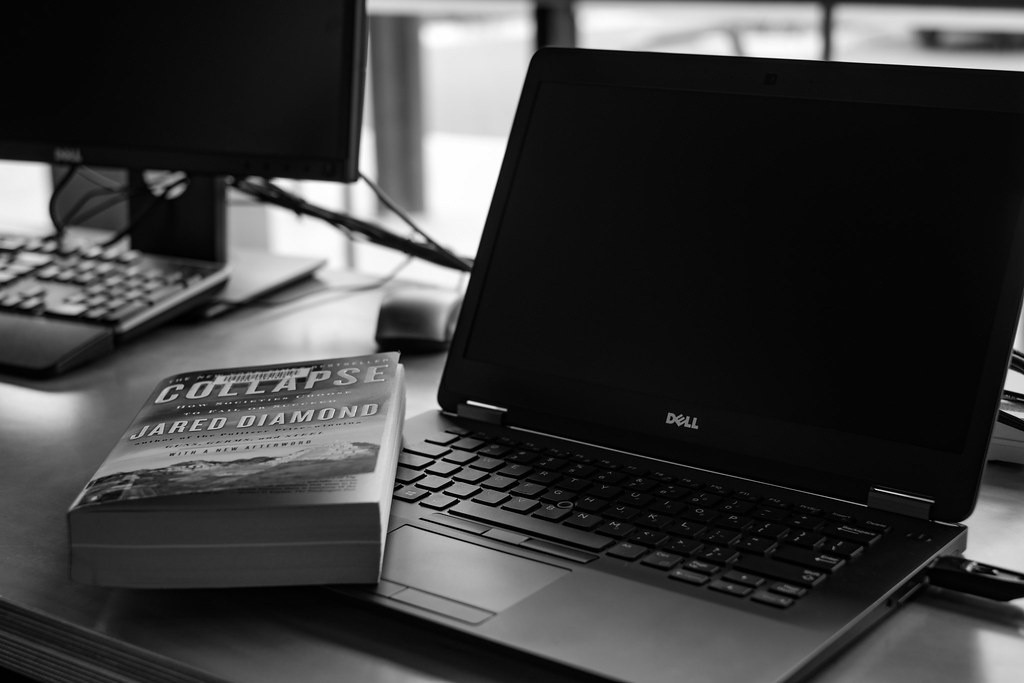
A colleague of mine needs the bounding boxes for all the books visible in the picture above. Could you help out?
[68,353,405,588]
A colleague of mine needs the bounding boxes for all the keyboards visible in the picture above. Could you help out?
[0,232,232,381]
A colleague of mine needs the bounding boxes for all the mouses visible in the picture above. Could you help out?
[374,283,465,351]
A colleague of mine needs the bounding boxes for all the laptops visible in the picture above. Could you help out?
[250,46,1024,683]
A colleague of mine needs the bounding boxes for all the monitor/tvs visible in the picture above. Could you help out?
[0,0,367,308]
